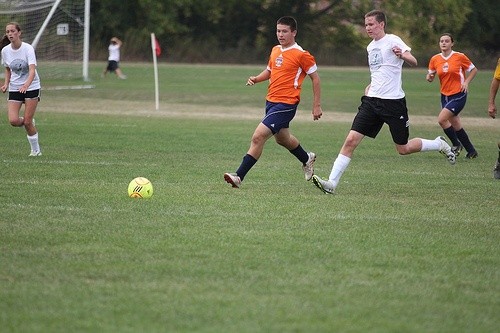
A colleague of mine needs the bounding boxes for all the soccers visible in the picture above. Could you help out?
[128,176,153,198]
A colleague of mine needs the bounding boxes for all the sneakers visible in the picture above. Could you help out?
[494,162,500,179]
[312,174,335,194]
[32,118,35,127]
[434,136,456,164]
[29,151,42,156]
[224,172,242,188]
[465,151,478,159]
[302,151,316,181]
[452,139,463,156]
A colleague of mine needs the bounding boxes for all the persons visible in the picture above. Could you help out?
[222,16,322,189]
[487,57,500,180]
[312,9,456,194]
[425,33,478,159]
[101,37,127,81]
[0,22,42,157]
